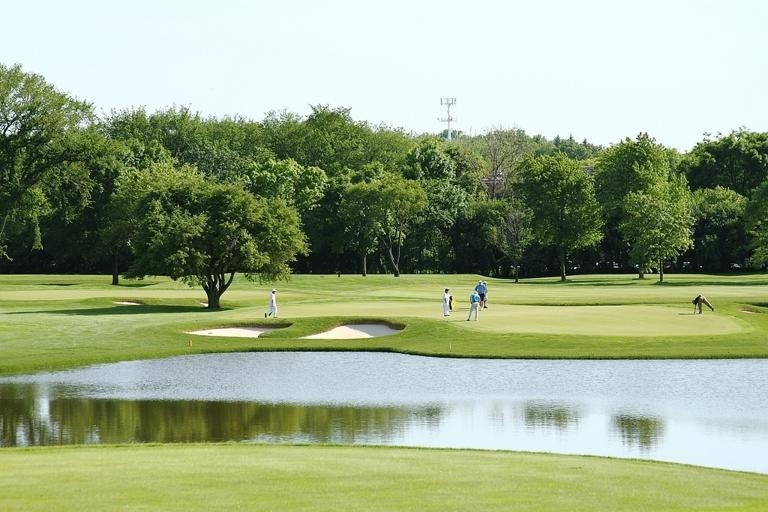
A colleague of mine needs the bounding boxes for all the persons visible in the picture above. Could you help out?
[692,294,714,314]
[483,282,488,308]
[442,289,451,316]
[265,289,278,318]
[466,292,480,321]
[475,280,485,311]
